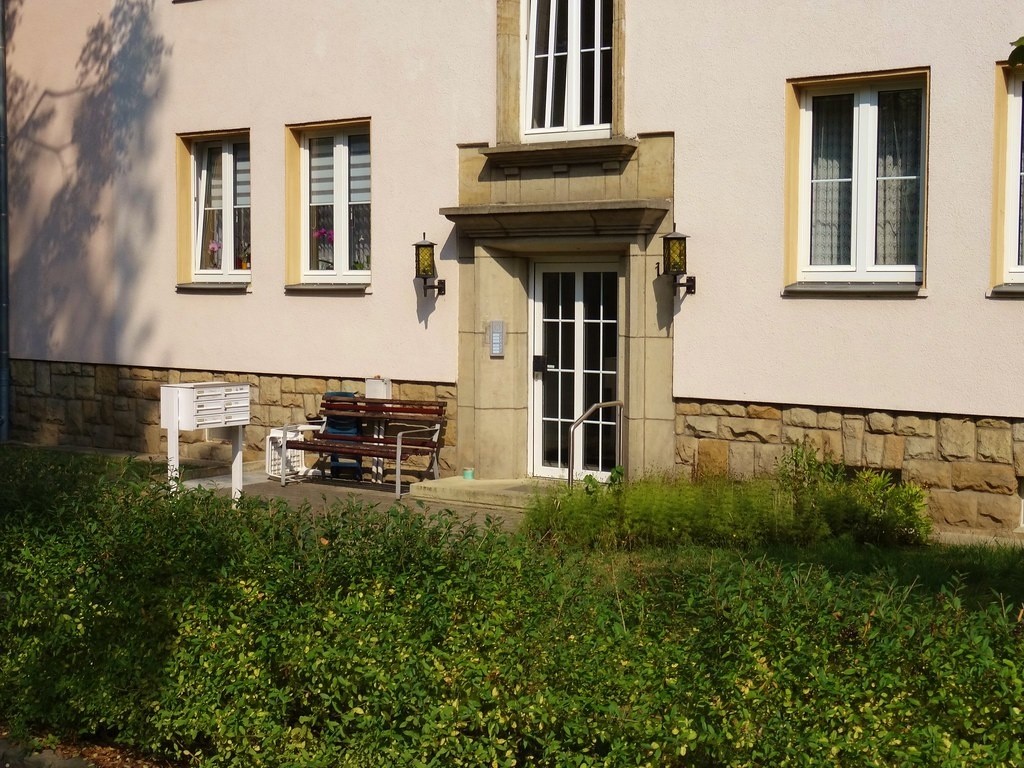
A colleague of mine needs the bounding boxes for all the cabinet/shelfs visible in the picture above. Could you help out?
[159,379,251,432]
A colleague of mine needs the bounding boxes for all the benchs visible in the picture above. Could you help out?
[281,395,446,501]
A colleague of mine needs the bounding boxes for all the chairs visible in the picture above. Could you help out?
[266,423,333,483]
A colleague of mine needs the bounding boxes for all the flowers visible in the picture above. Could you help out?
[206,241,221,269]
[310,229,334,271]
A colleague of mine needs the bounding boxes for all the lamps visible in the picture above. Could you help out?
[659,222,695,295]
[412,231,445,298]
